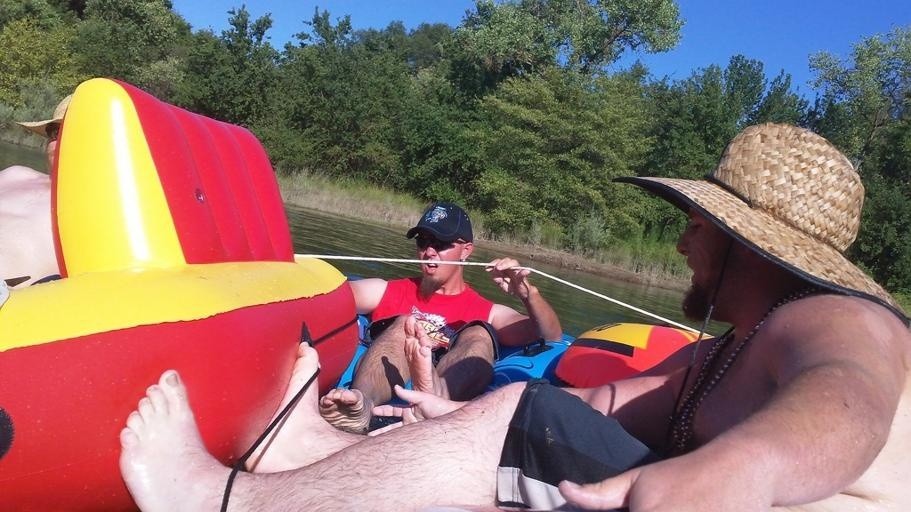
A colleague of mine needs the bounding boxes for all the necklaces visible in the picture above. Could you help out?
[668,284,830,452]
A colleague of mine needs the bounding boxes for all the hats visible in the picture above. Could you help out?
[613,124,910,323]
[16,94,71,138]
[407,204,473,250]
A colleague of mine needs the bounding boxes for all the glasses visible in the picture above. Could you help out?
[415,237,454,251]
[46,123,60,137]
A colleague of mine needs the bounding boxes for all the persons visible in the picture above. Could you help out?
[319,203,562,433]
[0,92,76,287]
[118,122,911,512]
